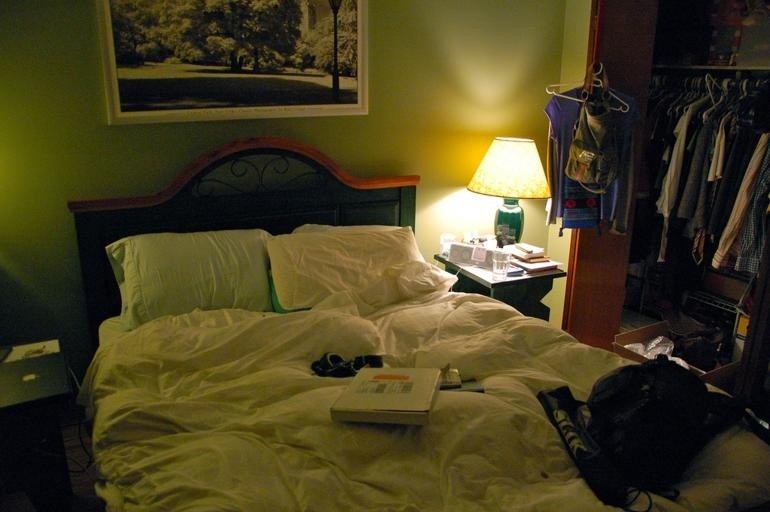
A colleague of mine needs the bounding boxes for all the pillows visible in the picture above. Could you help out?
[105,229,271,327]
[269,223,458,315]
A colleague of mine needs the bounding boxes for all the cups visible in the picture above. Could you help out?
[492,248,512,281]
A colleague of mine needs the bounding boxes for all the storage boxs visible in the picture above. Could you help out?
[610,311,743,395]
[1,339,70,408]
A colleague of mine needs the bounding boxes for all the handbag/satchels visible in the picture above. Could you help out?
[668,327,725,370]
[565,100,626,194]
[585,354,746,492]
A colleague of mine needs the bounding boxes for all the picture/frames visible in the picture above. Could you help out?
[98,0,370,128]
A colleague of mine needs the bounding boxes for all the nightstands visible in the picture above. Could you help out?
[434,245,567,321]
[0,340,87,512]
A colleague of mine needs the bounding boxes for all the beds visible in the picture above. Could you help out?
[67,135,768,512]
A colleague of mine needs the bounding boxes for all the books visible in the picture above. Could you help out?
[331,365,485,427]
[504,242,558,274]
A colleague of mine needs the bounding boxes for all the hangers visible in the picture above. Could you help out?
[544,65,769,126]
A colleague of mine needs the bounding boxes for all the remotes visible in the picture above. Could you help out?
[495,267,524,276]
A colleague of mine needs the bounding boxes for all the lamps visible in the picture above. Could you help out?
[466,136,554,247]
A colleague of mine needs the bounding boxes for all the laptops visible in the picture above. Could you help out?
[0,352,69,412]
